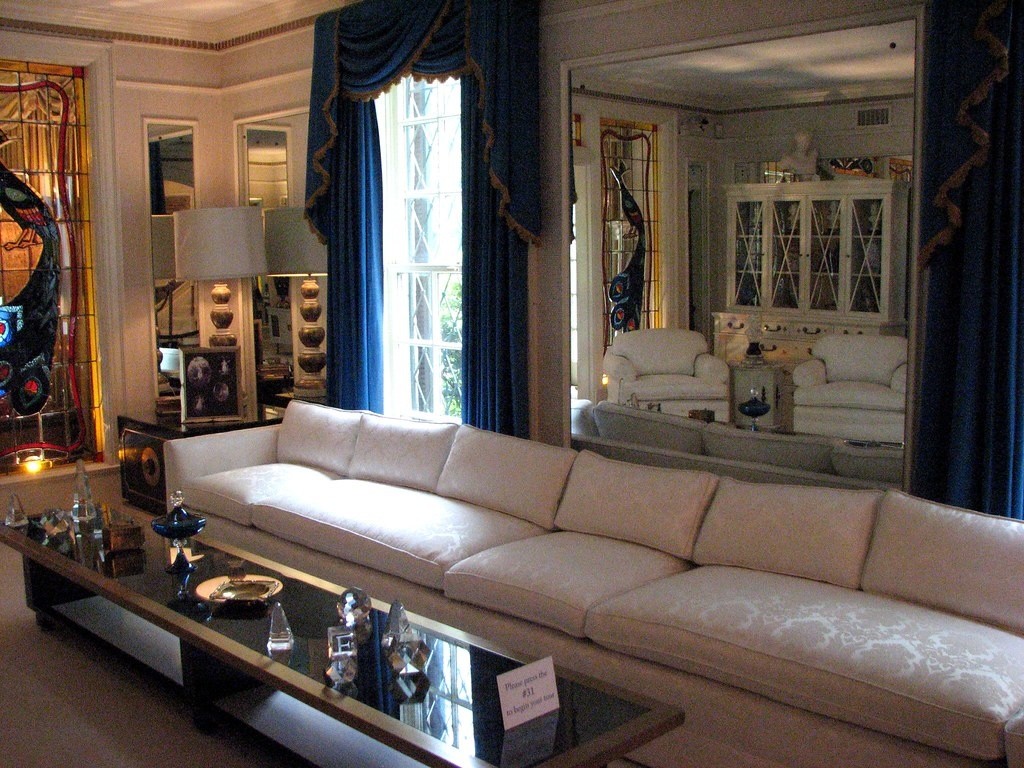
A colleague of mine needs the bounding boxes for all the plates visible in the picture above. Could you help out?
[196,574,283,602]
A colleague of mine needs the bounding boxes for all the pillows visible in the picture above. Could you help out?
[277,398,369,477]
[693,475,885,589]
[553,449,718,561]
[861,488,1024,637]
[348,413,459,494]
[571,399,903,482]
[436,424,577,530]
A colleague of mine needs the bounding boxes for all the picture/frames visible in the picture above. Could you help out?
[177,346,244,422]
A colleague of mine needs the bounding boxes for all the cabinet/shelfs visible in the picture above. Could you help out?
[712,177,910,369]
[734,364,778,427]
[117,405,283,516]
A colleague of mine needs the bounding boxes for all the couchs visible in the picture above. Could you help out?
[603,327,730,422]
[793,335,907,444]
[162,422,1024,768]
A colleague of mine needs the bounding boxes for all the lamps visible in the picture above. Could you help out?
[174,205,268,347]
[150,215,174,280]
[742,314,764,365]
[264,208,327,397]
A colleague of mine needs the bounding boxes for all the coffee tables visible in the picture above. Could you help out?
[0,500,686,768]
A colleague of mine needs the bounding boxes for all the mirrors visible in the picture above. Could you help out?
[234,106,308,412]
[143,118,201,403]
[561,3,925,492]
